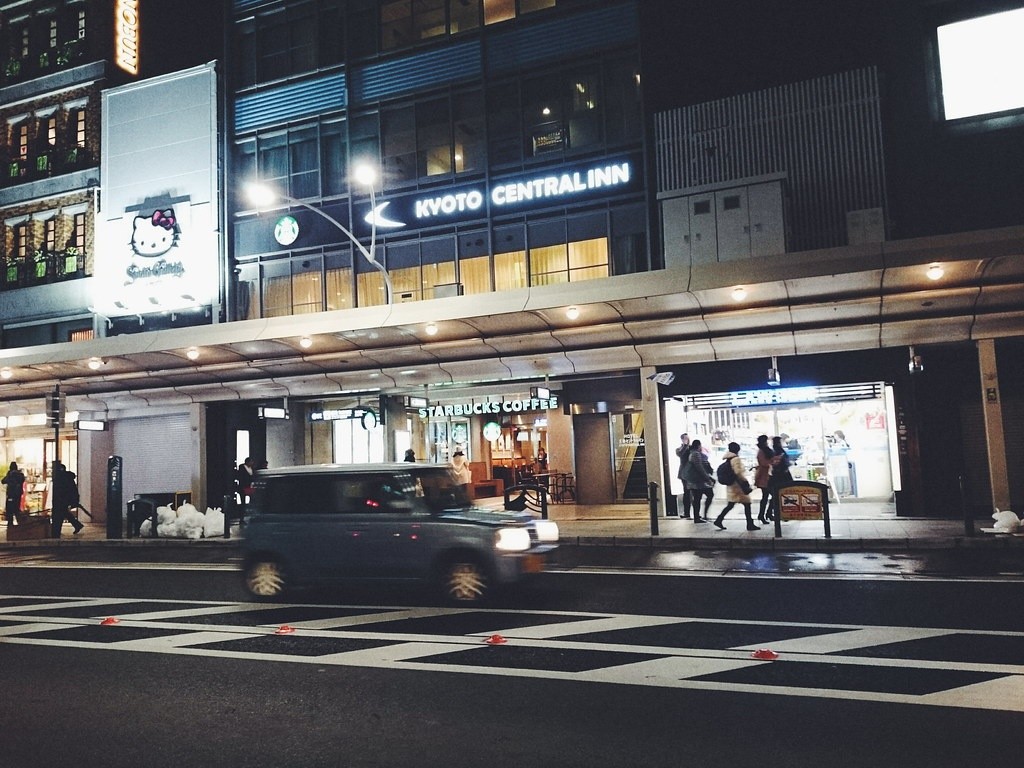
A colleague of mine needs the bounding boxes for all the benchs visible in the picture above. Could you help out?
[466,462,505,500]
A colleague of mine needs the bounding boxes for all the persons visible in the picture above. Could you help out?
[536,447,548,492]
[257,459,269,471]
[51,464,85,539]
[753,433,797,525]
[239,457,258,525]
[451,445,472,506]
[675,433,717,523]
[833,430,855,499]
[1,461,26,528]
[714,442,761,531]
[403,449,418,498]
[20,469,29,513]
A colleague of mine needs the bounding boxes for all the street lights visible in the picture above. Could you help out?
[241,155,395,305]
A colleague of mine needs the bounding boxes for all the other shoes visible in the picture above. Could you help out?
[72,524,84,535]
[695,518,706,523]
[680,514,691,518]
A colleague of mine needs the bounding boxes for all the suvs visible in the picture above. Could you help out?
[241,460,563,608]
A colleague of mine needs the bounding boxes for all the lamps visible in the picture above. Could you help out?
[909,345,924,375]
[402,384,430,411]
[530,374,552,400]
[73,409,110,433]
[257,396,290,421]
[767,357,781,386]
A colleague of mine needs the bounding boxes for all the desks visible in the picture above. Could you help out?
[534,472,572,504]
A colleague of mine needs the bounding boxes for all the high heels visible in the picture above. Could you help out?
[758,513,770,525]
[765,511,776,523]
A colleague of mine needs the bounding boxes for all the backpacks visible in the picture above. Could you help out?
[717,455,737,486]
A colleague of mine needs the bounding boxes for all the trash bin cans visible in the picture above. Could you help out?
[134,491,192,537]
[894,491,912,516]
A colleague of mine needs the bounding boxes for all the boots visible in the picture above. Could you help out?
[715,515,727,529]
[747,519,761,531]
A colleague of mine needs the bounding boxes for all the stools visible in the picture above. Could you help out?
[515,469,576,504]
